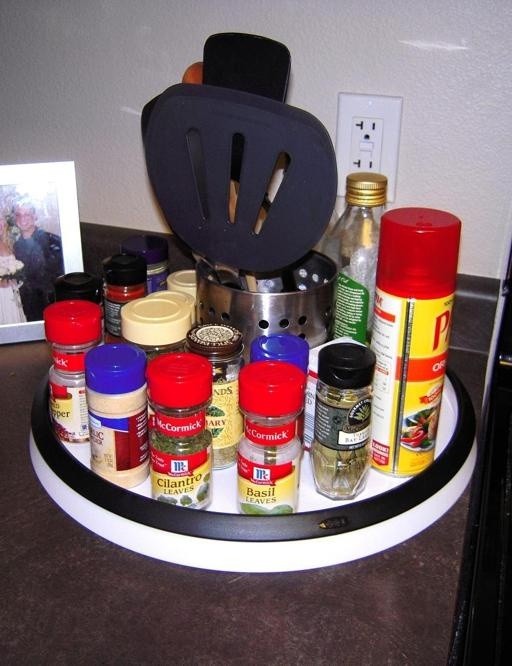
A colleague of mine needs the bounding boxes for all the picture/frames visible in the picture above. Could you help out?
[0,158,84,345]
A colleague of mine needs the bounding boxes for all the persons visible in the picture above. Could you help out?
[0,212,24,326]
[12,201,64,322]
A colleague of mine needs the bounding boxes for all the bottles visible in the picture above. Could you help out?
[321,171,389,345]
[50,269,107,344]
[101,233,200,355]
[370,206,463,480]
[42,300,104,444]
[314,206,339,255]
[144,351,212,512]
[84,341,150,490]
[237,332,377,516]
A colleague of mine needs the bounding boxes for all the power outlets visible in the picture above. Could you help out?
[334,90,403,203]
